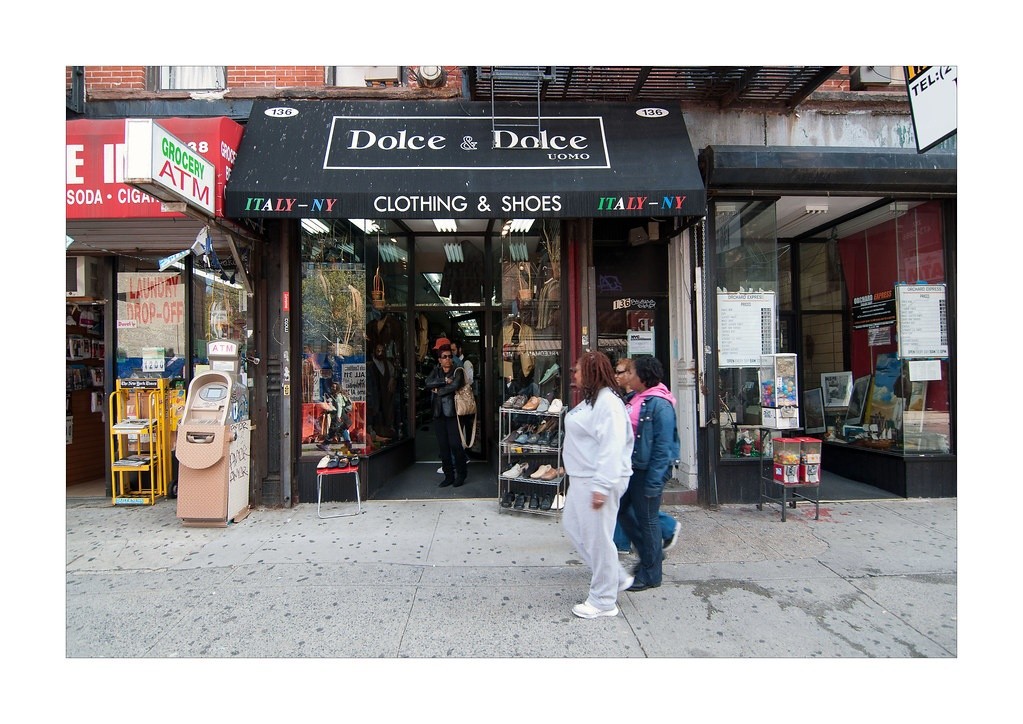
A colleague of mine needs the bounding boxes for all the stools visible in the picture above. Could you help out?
[316,461,361,518]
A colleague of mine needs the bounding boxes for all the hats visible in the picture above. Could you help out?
[432,337,451,350]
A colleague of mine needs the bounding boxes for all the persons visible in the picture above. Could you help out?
[450,342,475,456]
[424,344,469,488]
[432,338,470,475]
[621,350,680,594]
[612,356,682,558]
[372,343,398,437]
[558,349,635,619]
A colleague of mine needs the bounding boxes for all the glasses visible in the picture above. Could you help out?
[439,354,454,359]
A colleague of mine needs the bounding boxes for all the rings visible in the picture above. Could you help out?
[598,499,604,504]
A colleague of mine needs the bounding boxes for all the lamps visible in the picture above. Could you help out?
[301,217,536,235]
[819,205,908,239]
[332,239,530,263]
[746,205,829,240]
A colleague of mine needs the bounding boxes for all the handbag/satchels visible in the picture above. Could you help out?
[452,366,477,449]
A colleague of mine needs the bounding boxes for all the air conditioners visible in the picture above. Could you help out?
[66,255,104,298]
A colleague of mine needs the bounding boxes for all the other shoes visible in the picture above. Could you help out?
[439,472,452,486]
[501,395,567,512]
[453,472,468,487]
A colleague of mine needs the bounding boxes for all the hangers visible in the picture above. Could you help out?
[449,235,483,254]
[501,255,560,333]
[300,262,362,303]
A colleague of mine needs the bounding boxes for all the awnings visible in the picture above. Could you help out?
[223,93,709,222]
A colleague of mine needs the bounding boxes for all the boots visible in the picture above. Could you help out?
[316,439,332,450]
[340,439,352,449]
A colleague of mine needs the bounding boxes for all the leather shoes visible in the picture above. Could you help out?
[624,577,663,591]
[338,457,348,467]
[350,454,359,466]
[327,456,338,468]
[317,455,329,469]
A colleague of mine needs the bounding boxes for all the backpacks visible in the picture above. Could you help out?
[341,393,353,412]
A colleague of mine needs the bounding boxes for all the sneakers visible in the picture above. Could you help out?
[661,521,682,552]
[573,598,618,620]
[619,575,635,593]
[615,544,634,555]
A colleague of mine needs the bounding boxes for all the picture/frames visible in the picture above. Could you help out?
[801,387,827,435]
[716,292,777,368]
[819,370,854,408]
[841,373,872,427]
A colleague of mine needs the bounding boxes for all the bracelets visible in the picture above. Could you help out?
[445,376,448,385]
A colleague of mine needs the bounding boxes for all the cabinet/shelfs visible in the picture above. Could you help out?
[398,341,434,430]
[758,426,820,522]
[498,405,569,521]
[108,377,179,508]
